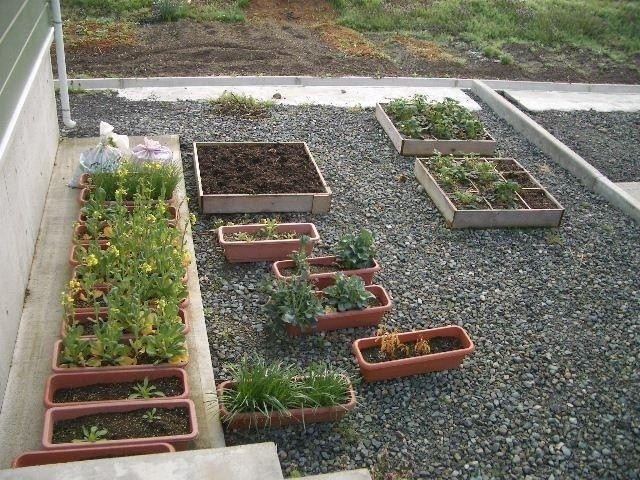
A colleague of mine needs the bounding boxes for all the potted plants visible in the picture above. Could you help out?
[373,90,567,226]
[39,372,198,442]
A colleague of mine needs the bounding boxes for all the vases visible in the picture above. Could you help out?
[12,444,175,467]
[191,140,333,215]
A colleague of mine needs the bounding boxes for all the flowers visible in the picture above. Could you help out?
[52,168,189,369]
